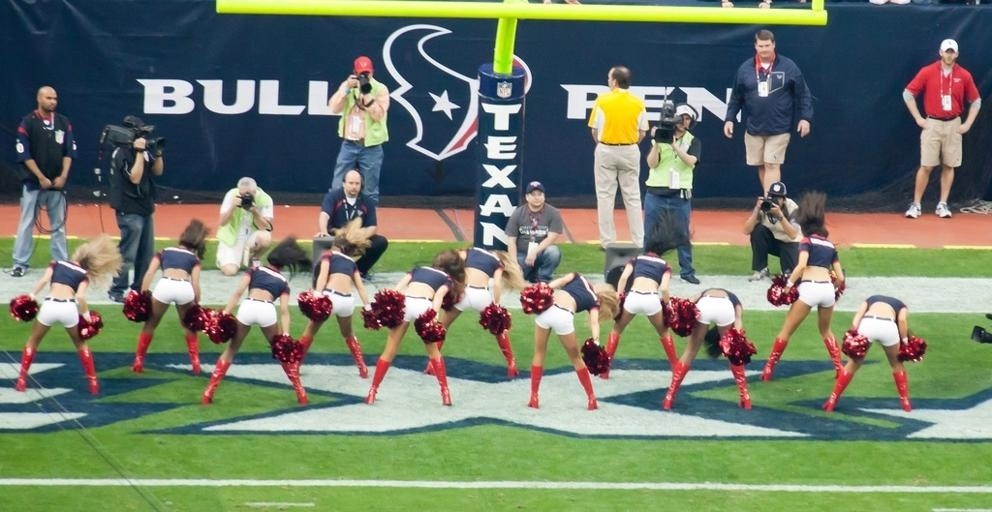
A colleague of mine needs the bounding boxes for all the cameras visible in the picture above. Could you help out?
[352,73,372,94]
[761,198,777,211]
[236,192,255,210]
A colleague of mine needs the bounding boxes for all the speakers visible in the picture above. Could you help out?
[313,237,334,291]
[604,243,640,292]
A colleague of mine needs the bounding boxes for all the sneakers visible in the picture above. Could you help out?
[11,268,24,278]
[749,268,770,281]
[936,202,952,218]
[905,205,921,218]
[109,292,127,304]
[681,272,699,284]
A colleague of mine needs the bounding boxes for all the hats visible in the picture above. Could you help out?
[355,57,373,75]
[941,39,958,52]
[675,105,693,117]
[525,181,544,193]
[768,181,787,198]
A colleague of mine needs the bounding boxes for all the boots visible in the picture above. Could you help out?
[367,359,390,403]
[280,336,308,403]
[346,336,368,377]
[577,368,598,410]
[600,334,618,379]
[662,337,689,411]
[894,371,912,412]
[760,338,787,380]
[528,365,542,408]
[496,331,519,378]
[78,347,100,394]
[17,346,34,391]
[729,362,751,411]
[204,359,230,404]
[424,338,452,406]
[187,335,200,374]
[132,334,151,372]
[822,337,853,410]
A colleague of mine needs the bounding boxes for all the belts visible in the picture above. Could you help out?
[803,279,829,284]
[631,289,658,294]
[555,303,573,314]
[324,286,352,298]
[246,296,273,304]
[469,284,488,289]
[44,296,75,302]
[605,143,629,146]
[407,295,431,300]
[163,276,191,282]
[929,116,955,121]
[863,314,889,321]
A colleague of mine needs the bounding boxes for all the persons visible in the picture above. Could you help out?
[760,197,845,383]
[643,102,702,285]
[135,219,209,374]
[723,29,815,199]
[504,180,563,284]
[587,66,651,252]
[742,181,804,281]
[298,220,533,405]
[9,86,80,277]
[821,295,910,410]
[329,56,390,211]
[722,0,772,8]
[204,235,314,405]
[902,38,982,219]
[9,232,121,395]
[314,170,389,283]
[107,114,163,303]
[215,177,273,276]
[527,270,623,411]
[600,250,752,410]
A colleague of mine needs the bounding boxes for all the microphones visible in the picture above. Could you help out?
[685,127,694,133]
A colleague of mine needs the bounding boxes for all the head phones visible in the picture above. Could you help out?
[675,102,699,130]
[343,169,365,190]
